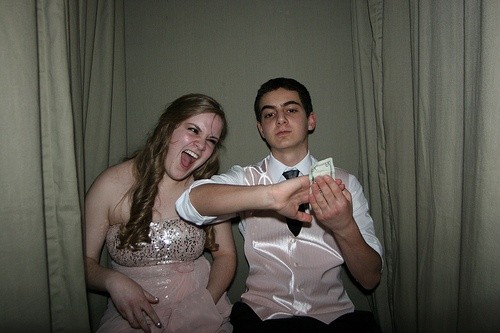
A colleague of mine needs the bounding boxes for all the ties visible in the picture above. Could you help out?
[282,170,305,236]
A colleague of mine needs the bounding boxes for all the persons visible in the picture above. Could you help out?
[175,78,384,333]
[81,94,237,333]
